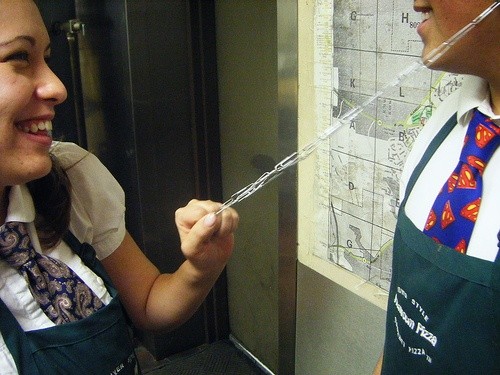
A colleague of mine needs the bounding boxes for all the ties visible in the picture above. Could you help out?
[422,106,500,256]
[0,222,105,326]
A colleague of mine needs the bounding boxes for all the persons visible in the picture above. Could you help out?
[1,1,243,374]
[371,0,500,375]
